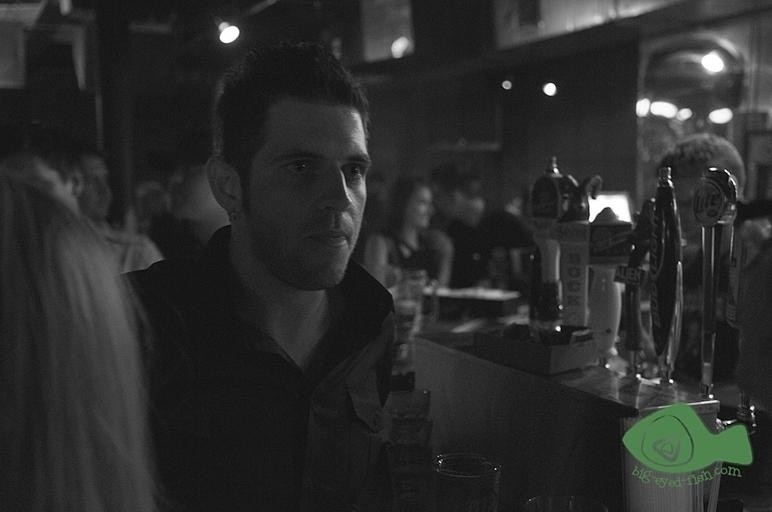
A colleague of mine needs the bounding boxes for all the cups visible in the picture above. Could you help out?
[523,493,608,511]
[389,269,427,344]
[429,451,502,511]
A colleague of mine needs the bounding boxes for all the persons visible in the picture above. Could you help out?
[5,138,532,326]
[115,44,439,510]
[657,134,769,397]
[4,150,165,510]
